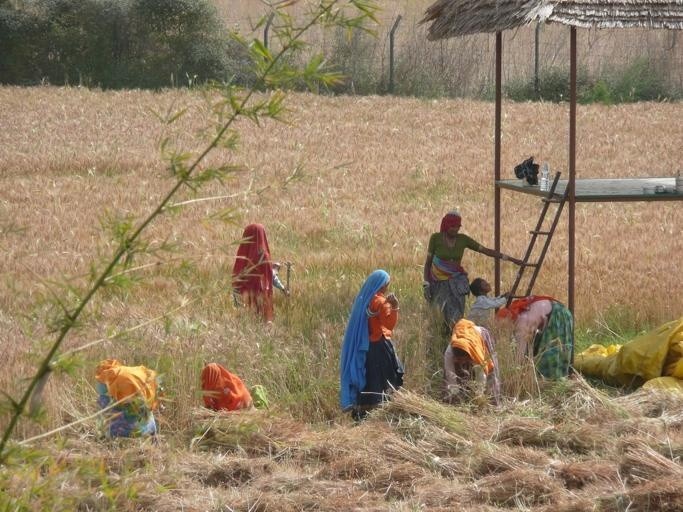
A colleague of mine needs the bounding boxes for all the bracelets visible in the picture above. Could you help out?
[501,254,509,261]
[422,280,431,290]
[390,306,400,311]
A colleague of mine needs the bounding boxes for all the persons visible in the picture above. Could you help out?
[231,221,292,320]
[464,276,511,325]
[421,209,527,332]
[95,358,161,439]
[492,293,573,379]
[200,361,255,411]
[441,317,499,409]
[336,267,403,420]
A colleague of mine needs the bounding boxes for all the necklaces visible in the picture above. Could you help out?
[445,235,457,248]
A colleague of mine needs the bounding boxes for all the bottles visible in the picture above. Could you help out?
[539,163,549,192]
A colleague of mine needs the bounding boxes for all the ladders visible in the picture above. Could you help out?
[507,172,570,308]
